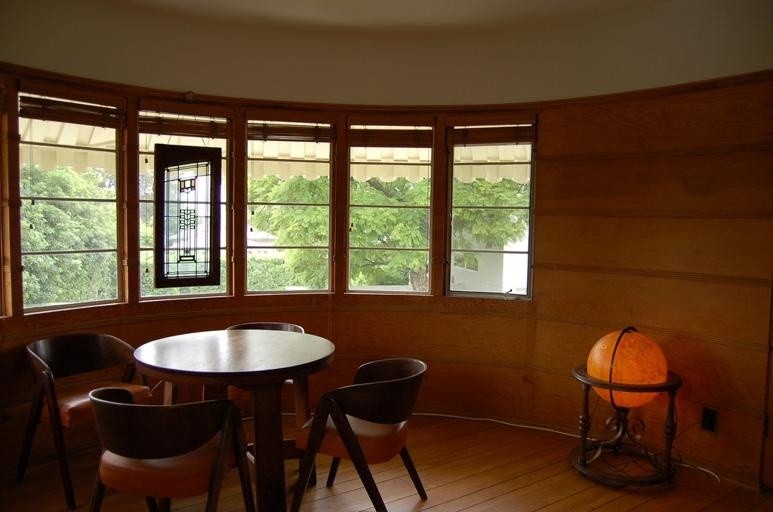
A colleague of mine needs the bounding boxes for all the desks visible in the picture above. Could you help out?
[133,330,335,511]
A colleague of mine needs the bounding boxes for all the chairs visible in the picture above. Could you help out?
[13,331,145,511]
[291,357,428,512]
[205,322,318,470]
[87,387,253,512]
